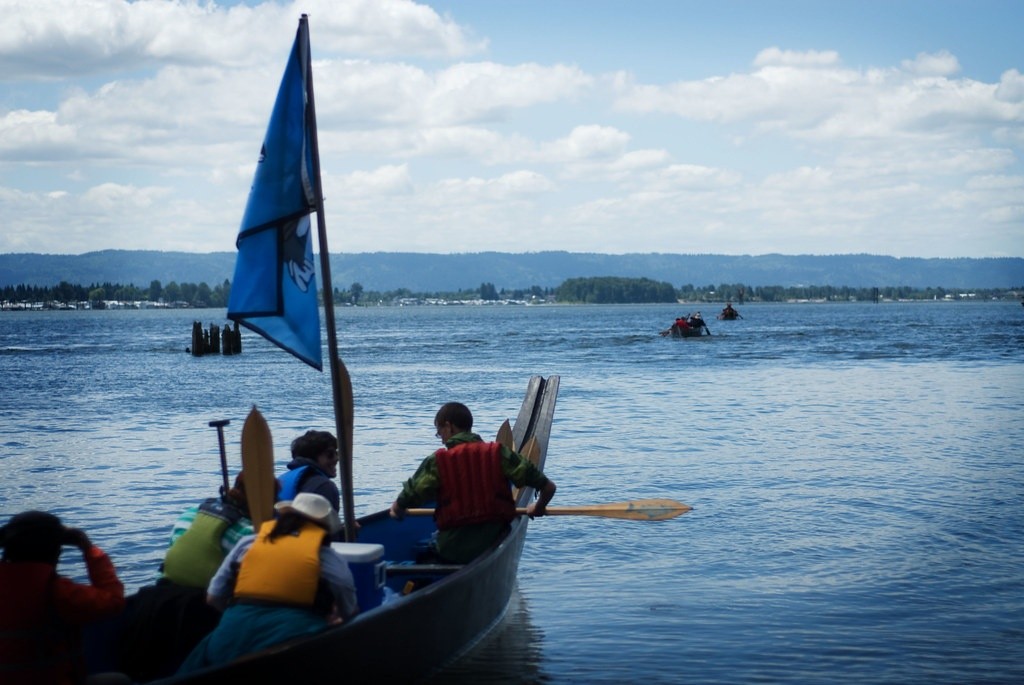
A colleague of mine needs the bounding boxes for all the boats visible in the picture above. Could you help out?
[671,310,706,338]
[62,10,562,684]
[720,303,738,320]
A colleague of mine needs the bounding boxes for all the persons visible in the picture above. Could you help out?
[157,430,357,673]
[674,316,688,328]
[694,311,702,320]
[0,511,126,685]
[388,401,557,575]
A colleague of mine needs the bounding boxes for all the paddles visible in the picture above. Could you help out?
[401,499,694,521]
[211,419,239,494]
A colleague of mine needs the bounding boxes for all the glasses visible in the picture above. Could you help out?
[435,424,448,438]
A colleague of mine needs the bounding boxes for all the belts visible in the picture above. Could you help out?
[226,597,314,611]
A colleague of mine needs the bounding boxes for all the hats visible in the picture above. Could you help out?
[274,492,341,535]
[230,471,281,503]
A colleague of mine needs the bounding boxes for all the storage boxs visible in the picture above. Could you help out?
[330,542,387,612]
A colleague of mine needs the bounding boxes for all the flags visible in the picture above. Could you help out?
[227,13,325,372]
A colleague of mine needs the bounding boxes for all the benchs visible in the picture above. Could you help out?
[386,564,463,575]
